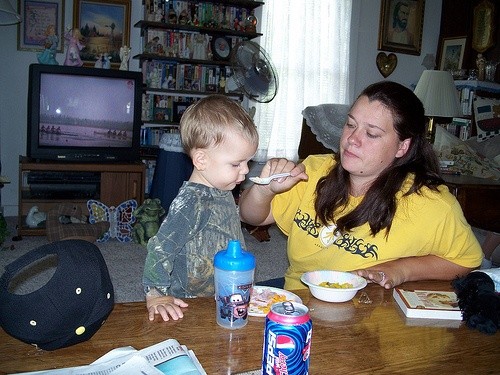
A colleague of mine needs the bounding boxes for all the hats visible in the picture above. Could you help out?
[0,239,115,351]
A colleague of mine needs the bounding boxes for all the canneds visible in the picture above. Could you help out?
[261,300,312,375]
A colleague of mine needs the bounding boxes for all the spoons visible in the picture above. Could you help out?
[249,173,291,185]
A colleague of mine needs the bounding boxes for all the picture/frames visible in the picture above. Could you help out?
[72,0,133,69]
[378,0,426,56]
[440,35,469,72]
[16,0,65,53]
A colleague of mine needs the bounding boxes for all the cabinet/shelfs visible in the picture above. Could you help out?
[16,161,147,238]
[133,0,265,201]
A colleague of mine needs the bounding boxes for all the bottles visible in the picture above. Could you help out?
[213,240,256,329]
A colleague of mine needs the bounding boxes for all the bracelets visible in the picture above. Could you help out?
[143,286,156,296]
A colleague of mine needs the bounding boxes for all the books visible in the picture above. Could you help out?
[142,60,246,94]
[141,93,241,123]
[140,125,181,148]
[145,27,249,61]
[439,117,471,142]
[458,88,475,115]
[147,2,255,32]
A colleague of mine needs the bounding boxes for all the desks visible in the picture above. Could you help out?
[442,173,500,217]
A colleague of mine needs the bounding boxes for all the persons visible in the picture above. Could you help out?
[37,24,58,65]
[141,95,259,321]
[237,82,485,292]
[63,28,85,66]
[41,125,61,134]
[107,128,128,137]
[476,53,486,81]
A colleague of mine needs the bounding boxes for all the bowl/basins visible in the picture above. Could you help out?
[300,269,368,303]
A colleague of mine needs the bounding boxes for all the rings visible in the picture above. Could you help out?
[371,271,385,283]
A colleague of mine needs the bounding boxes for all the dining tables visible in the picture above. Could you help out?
[0,280,500,375]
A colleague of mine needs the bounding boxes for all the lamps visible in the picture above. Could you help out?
[414,68,462,140]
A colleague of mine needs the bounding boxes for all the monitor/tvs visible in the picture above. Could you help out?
[27,64,144,165]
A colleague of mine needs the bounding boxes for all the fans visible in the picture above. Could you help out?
[230,40,277,111]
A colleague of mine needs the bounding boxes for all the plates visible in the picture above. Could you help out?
[247,285,303,318]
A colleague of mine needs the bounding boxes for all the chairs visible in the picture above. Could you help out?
[298,103,348,158]
